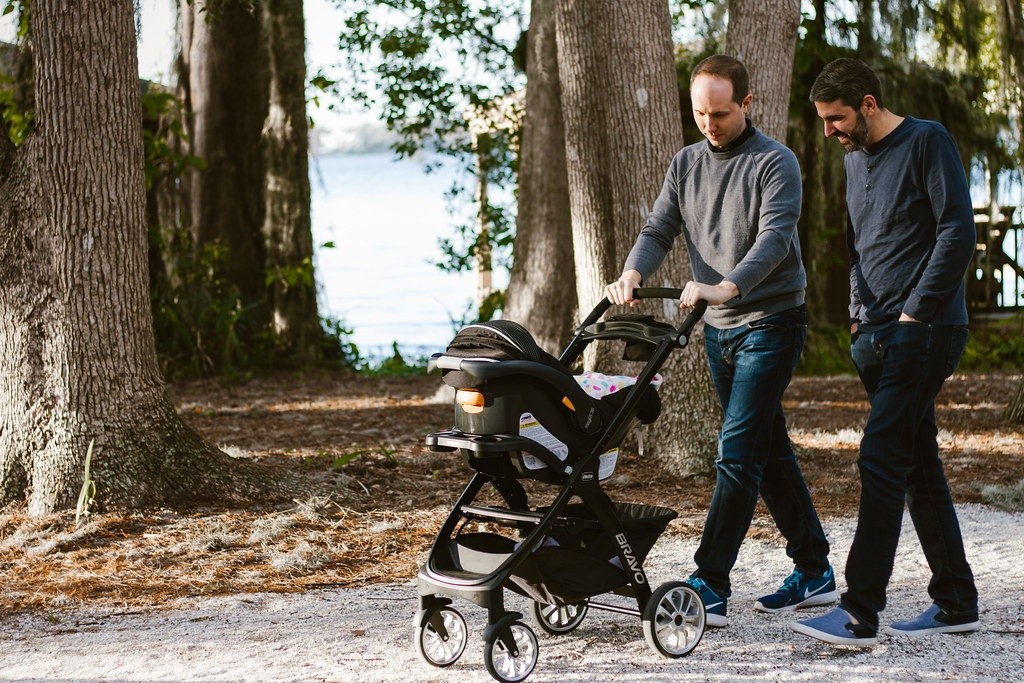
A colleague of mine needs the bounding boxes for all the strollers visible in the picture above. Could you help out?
[410,284,707,683]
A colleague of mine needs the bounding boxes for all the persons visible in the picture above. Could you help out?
[788,57,981,645]
[604,54,839,627]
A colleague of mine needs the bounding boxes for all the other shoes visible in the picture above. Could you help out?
[888,604,981,635]
[791,608,878,645]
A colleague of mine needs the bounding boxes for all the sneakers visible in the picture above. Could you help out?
[754,567,837,613]
[667,579,727,627]
